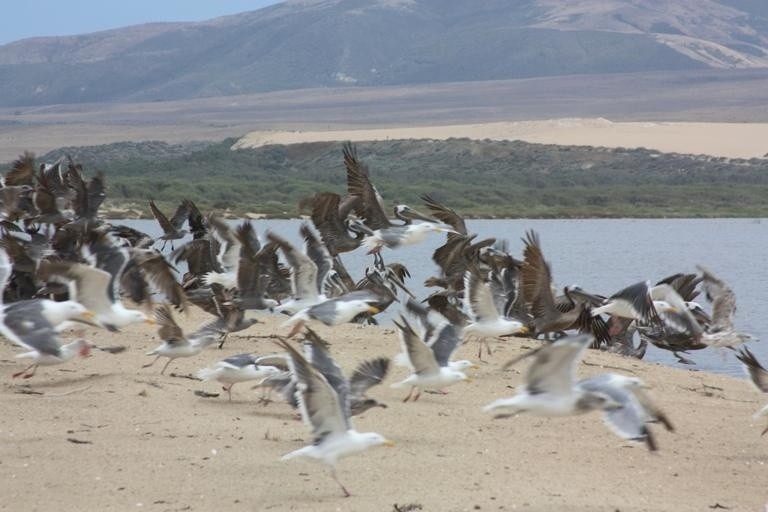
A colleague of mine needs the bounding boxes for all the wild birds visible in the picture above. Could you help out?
[0,139,768,497]
